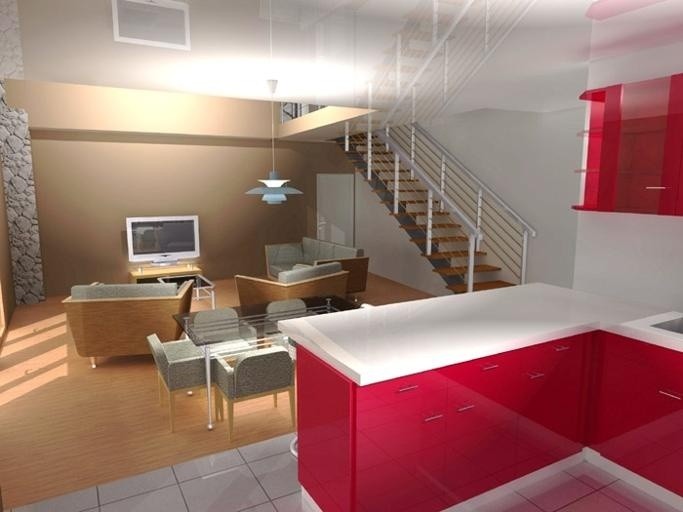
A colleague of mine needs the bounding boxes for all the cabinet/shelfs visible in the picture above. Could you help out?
[290,346,522,512]
[521,335,585,476]
[569,69,683,219]
[586,333,683,495]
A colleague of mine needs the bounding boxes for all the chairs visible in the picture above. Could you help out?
[59,279,196,369]
[144,298,316,444]
[234,262,352,305]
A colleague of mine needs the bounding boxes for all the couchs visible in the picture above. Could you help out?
[263,235,367,298]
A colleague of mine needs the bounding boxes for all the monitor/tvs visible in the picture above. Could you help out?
[126,215,202,267]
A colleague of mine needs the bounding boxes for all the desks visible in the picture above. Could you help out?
[126,264,203,285]
[170,293,361,432]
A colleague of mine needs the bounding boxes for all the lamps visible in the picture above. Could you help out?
[243,0,304,207]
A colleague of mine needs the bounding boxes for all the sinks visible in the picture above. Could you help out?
[650,315,682,337]
